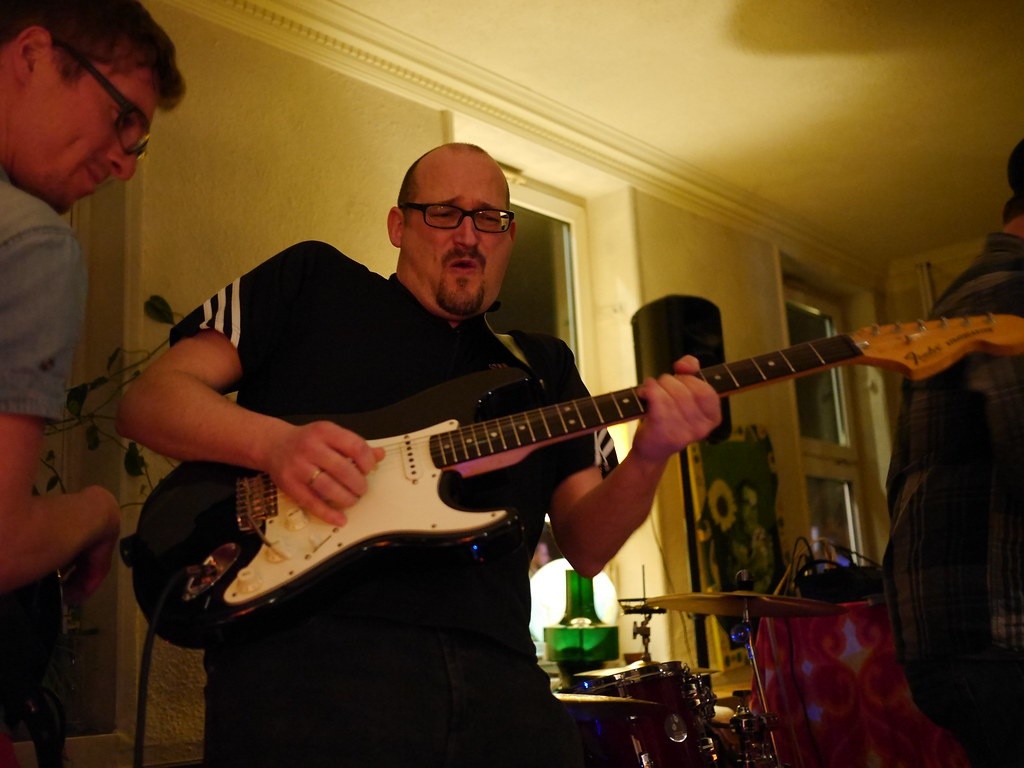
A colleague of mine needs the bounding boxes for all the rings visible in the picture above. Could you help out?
[306,467,321,485]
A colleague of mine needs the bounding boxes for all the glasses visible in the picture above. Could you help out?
[50,39,153,165]
[398,202,515,233]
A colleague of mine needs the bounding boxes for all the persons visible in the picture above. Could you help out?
[0,0,185,768]
[115,143,723,768]
[882,136,1024,768]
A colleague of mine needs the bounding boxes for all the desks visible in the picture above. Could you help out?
[751,597,969,768]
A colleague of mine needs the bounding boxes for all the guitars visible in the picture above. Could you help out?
[127,301,1024,652]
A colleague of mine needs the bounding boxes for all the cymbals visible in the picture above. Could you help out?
[657,570,850,619]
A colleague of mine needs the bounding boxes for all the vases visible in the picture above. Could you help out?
[544,571,619,693]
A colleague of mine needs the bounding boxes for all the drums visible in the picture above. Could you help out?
[556,658,714,768]
